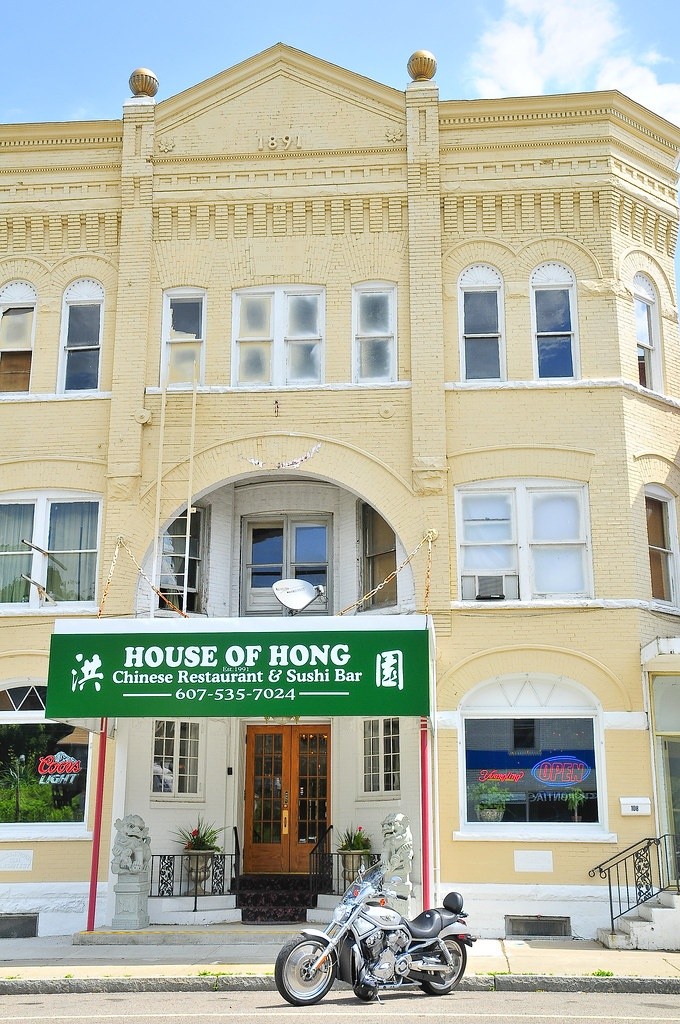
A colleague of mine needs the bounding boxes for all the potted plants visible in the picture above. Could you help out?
[564,787,588,822]
[467,781,514,822]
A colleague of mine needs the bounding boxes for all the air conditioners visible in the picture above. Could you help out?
[475,574,506,600]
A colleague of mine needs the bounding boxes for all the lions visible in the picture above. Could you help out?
[380,812,413,869]
[112,814,152,871]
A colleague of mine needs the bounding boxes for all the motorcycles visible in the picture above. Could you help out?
[275,861,478,1008]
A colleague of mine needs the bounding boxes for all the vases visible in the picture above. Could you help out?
[182,848,216,896]
[336,849,371,882]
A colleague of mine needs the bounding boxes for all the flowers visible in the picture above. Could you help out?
[167,813,232,853]
[333,821,374,861]
